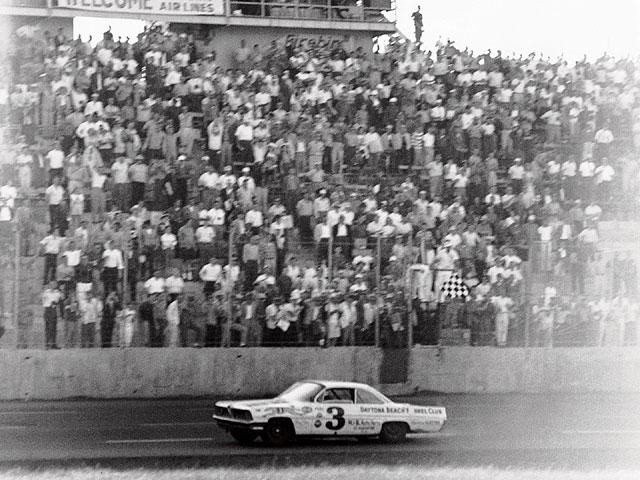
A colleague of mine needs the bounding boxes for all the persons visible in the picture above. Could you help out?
[0,6,639,347]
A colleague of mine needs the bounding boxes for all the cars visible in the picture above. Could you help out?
[211,378,448,446]
